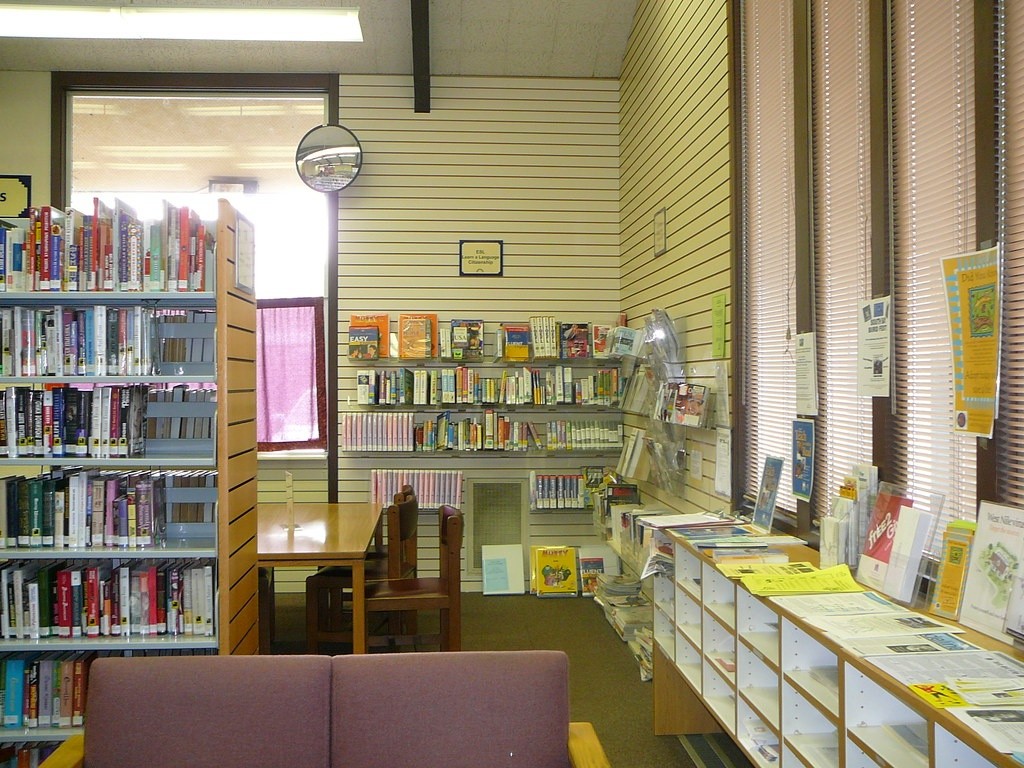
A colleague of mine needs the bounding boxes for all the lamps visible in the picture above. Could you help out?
[0,2,366,44]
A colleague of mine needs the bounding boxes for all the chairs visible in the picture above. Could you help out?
[317,484,416,635]
[364,504,465,655]
[258,566,275,655]
[304,495,419,655]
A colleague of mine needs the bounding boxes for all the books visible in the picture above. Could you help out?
[348,313,621,370]
[0,384,220,459]
[589,482,974,624]
[352,369,628,409]
[369,465,615,509]
[0,467,217,552]
[483,541,657,681]
[615,359,716,481]
[0,305,215,383]
[1,649,218,728]
[0,741,61,767]
[0,557,215,644]
[0,203,218,294]
[342,411,627,452]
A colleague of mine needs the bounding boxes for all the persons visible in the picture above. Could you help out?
[680,395,704,417]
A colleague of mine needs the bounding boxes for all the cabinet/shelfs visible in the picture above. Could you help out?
[0,195,261,768]
[342,353,627,457]
[594,536,654,679]
[652,514,1024,768]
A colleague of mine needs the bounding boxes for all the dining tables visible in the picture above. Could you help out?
[250,501,384,655]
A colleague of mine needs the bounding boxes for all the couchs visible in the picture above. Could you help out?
[39,649,610,768]
